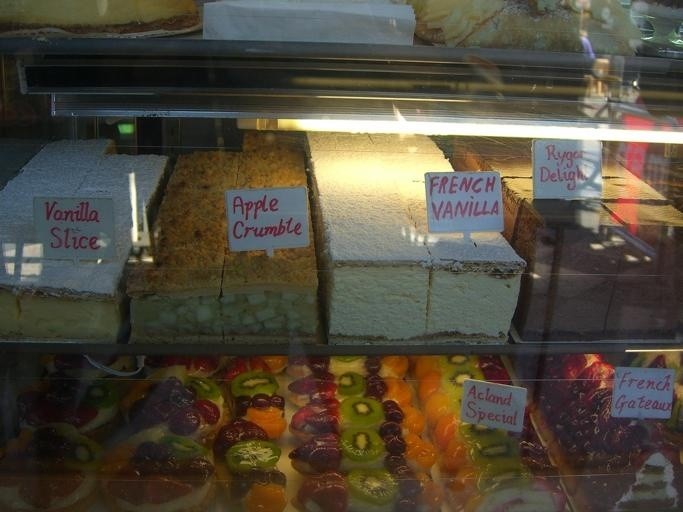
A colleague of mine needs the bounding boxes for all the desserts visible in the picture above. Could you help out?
[0,351,683,512]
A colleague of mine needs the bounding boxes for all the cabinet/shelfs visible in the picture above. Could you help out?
[0,38,683,512]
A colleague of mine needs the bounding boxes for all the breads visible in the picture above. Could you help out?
[0,130,683,345]
[0,0,683,58]
